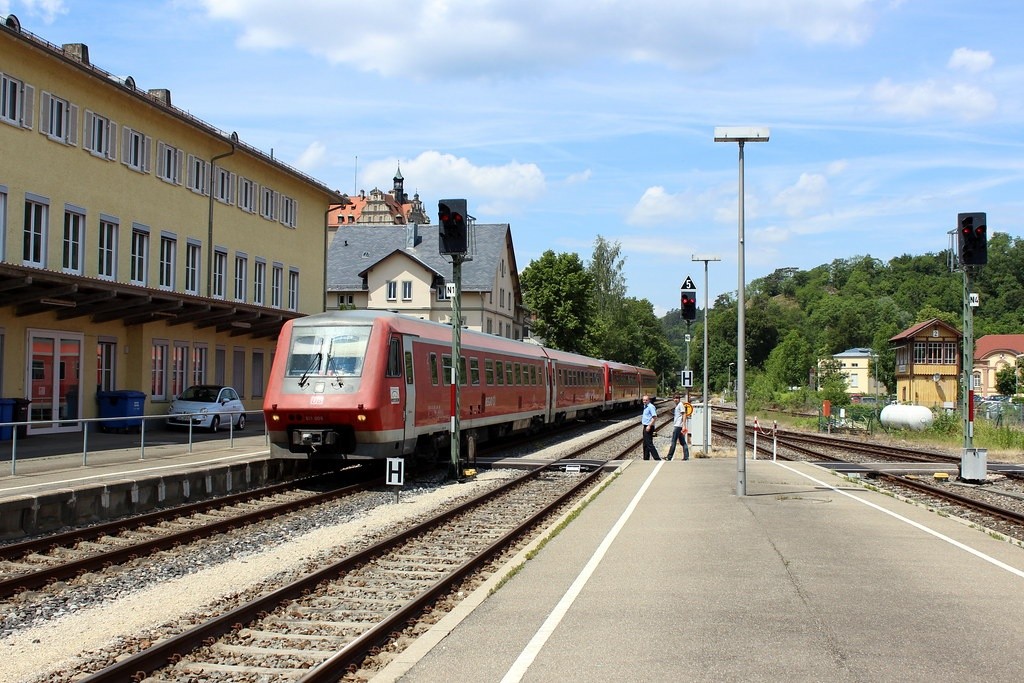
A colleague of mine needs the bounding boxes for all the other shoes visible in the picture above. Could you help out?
[682,458,689,461]
[663,456,671,461]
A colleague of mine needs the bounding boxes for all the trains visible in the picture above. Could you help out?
[264,310,658,466]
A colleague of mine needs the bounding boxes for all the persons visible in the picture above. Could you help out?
[662,395,689,461]
[642,396,661,460]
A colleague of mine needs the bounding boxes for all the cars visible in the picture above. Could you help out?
[848,394,875,406]
[976,394,1019,414]
[167,385,247,434]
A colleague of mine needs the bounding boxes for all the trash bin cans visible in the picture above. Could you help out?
[99,389,147,434]
[3,398,32,439]
[0,400,16,441]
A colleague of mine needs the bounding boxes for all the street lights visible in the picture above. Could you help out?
[1015,354,1024,394]
[715,126,769,499]
[692,254,723,453]
[874,353,880,403]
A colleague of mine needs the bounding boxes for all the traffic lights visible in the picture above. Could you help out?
[680,293,695,319]
[958,212,989,266]
[437,198,467,257]
